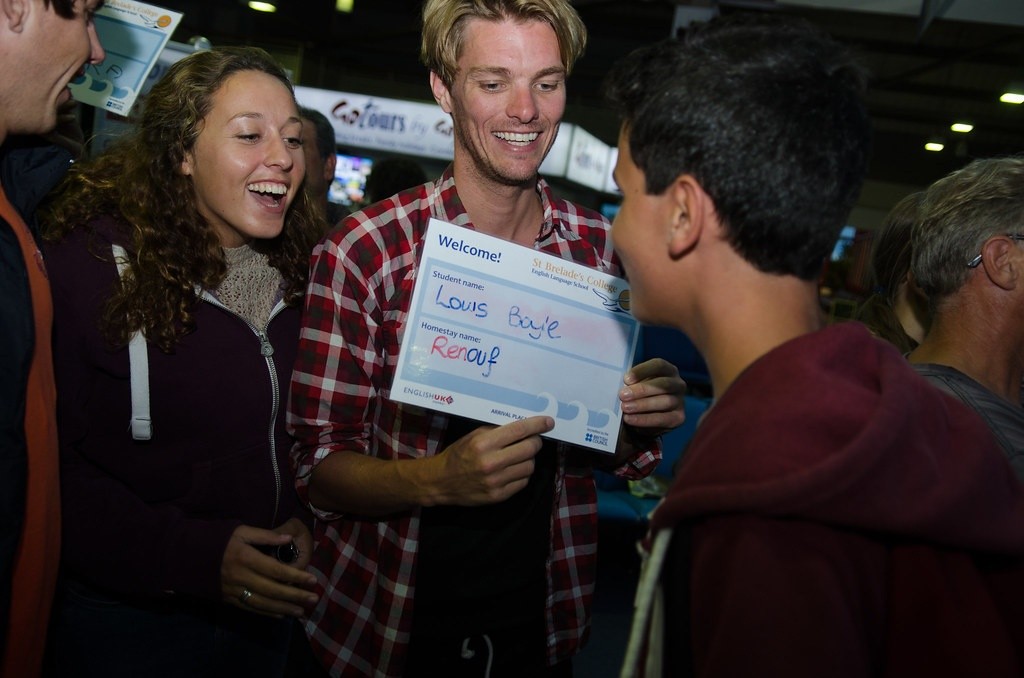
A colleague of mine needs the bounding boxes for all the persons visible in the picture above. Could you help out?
[865,191,938,362]
[360,158,425,202]
[297,105,349,227]
[905,154,1024,488]
[0,0,106,678]
[283,0,685,678]
[34,44,328,678]
[602,5,1024,678]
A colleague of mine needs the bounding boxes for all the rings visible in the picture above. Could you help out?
[239,589,252,603]
